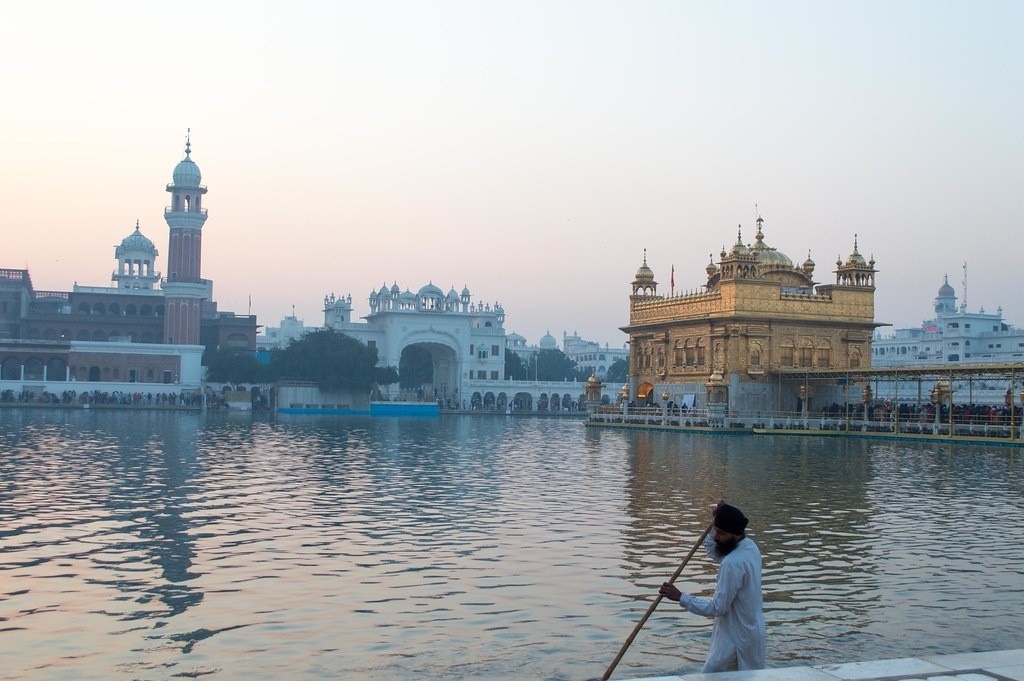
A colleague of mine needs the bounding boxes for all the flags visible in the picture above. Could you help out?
[670,267,674,288]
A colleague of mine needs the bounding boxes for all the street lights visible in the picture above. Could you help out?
[534,355,538,381]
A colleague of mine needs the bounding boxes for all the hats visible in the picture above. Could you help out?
[713,503,749,536]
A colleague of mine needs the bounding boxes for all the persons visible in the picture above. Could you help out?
[659,500,765,673]
[1,389,224,407]
[417,387,586,411]
[819,402,1024,426]
[620,400,696,417]
